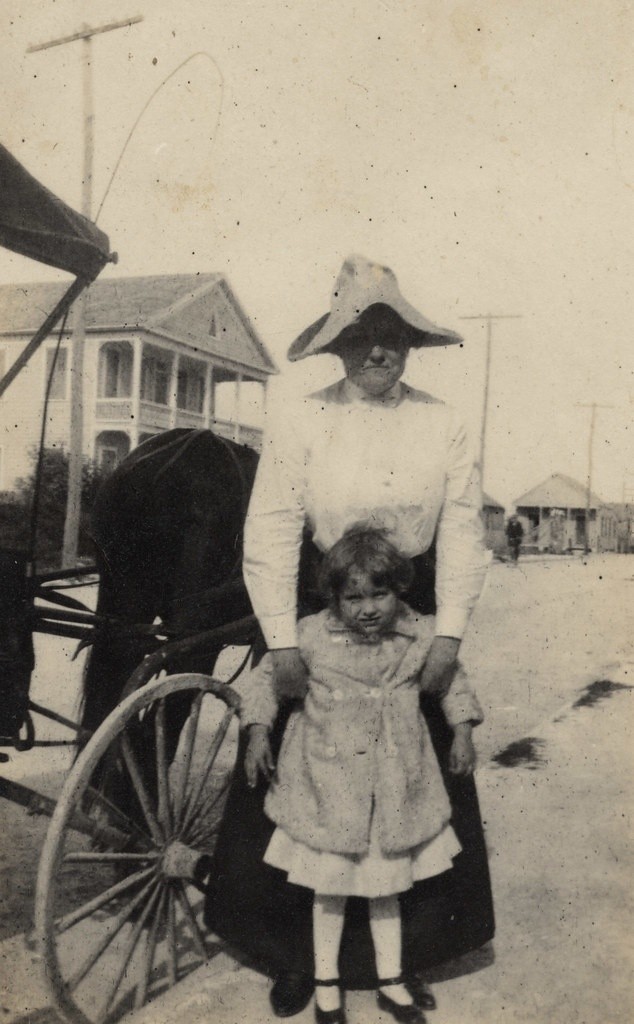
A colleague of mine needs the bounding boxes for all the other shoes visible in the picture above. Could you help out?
[270,977,314,1016]
[314,977,345,1024]
[376,977,426,1024]
[405,973,437,1010]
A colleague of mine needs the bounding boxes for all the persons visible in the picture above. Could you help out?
[236,519,486,1024]
[206,253,498,1019]
[504,514,523,564]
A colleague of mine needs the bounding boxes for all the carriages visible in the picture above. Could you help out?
[0,143,309,1024]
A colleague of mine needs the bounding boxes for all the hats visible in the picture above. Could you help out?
[287,255,465,363]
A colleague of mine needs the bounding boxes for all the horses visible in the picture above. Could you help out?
[76,422,328,930]
